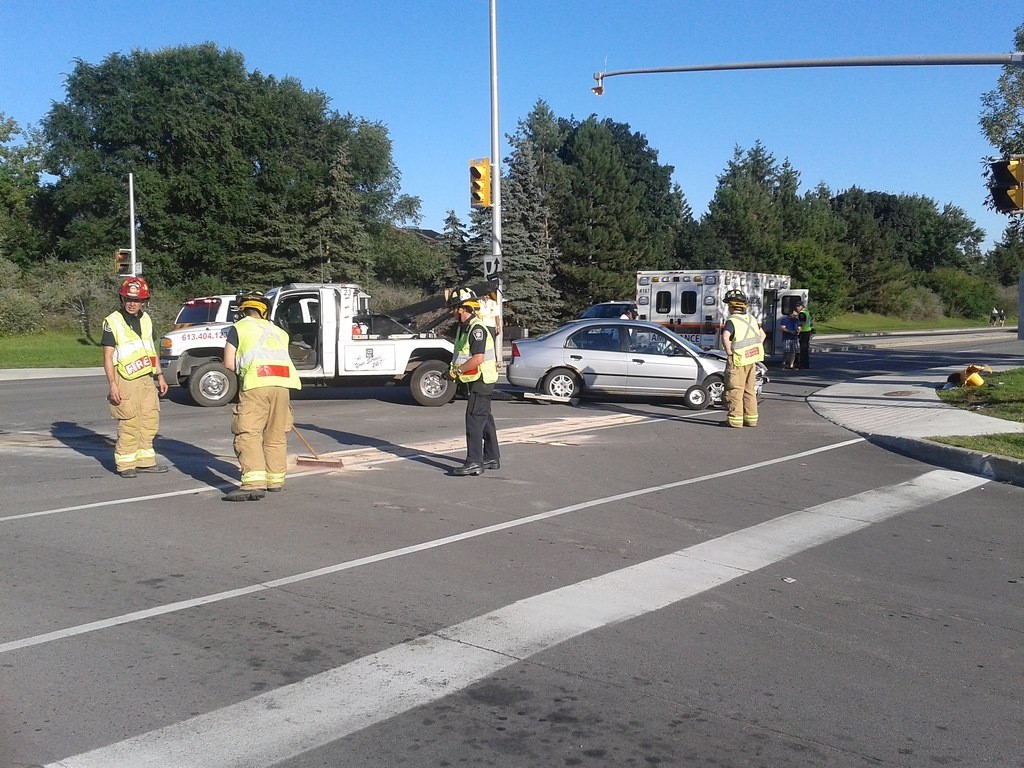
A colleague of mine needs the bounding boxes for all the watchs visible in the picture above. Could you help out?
[458,368,461,373]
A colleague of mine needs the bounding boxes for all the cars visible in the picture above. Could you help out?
[561,300,638,342]
[173,293,239,331]
[505,317,770,412]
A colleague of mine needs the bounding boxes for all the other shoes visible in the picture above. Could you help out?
[782,363,786,370]
[227,488,265,501]
[269,488,281,491]
[719,421,729,426]
[791,367,798,370]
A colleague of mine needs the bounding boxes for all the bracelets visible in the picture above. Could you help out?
[157,372,163,376]
[728,354,732,356]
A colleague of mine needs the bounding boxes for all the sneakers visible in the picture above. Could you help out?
[121,469,137,478]
[136,464,169,473]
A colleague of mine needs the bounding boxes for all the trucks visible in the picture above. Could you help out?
[635,269,815,364]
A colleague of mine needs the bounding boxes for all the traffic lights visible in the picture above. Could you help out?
[115,250,131,275]
[468,157,490,208]
[990,159,1024,210]
[959,365,992,388]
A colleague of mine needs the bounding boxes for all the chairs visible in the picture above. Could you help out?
[289,321,319,364]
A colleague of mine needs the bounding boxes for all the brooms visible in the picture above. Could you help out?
[291,425,345,467]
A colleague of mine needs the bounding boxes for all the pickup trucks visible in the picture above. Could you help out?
[159,283,461,407]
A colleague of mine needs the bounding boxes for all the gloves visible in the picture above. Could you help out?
[441,371,454,381]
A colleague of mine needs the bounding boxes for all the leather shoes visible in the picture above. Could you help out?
[453,462,485,475]
[484,459,500,469]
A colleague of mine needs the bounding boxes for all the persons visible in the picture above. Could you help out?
[990,307,999,327]
[222,297,302,501]
[796,301,813,369]
[101,278,168,478]
[619,309,639,320]
[781,310,800,368]
[721,290,766,428]
[440,288,500,476]
[999,309,1006,327]
[477,294,502,370]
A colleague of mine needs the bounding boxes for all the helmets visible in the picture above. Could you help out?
[239,291,270,317]
[118,276,151,300]
[722,289,749,307]
[447,287,480,309]
